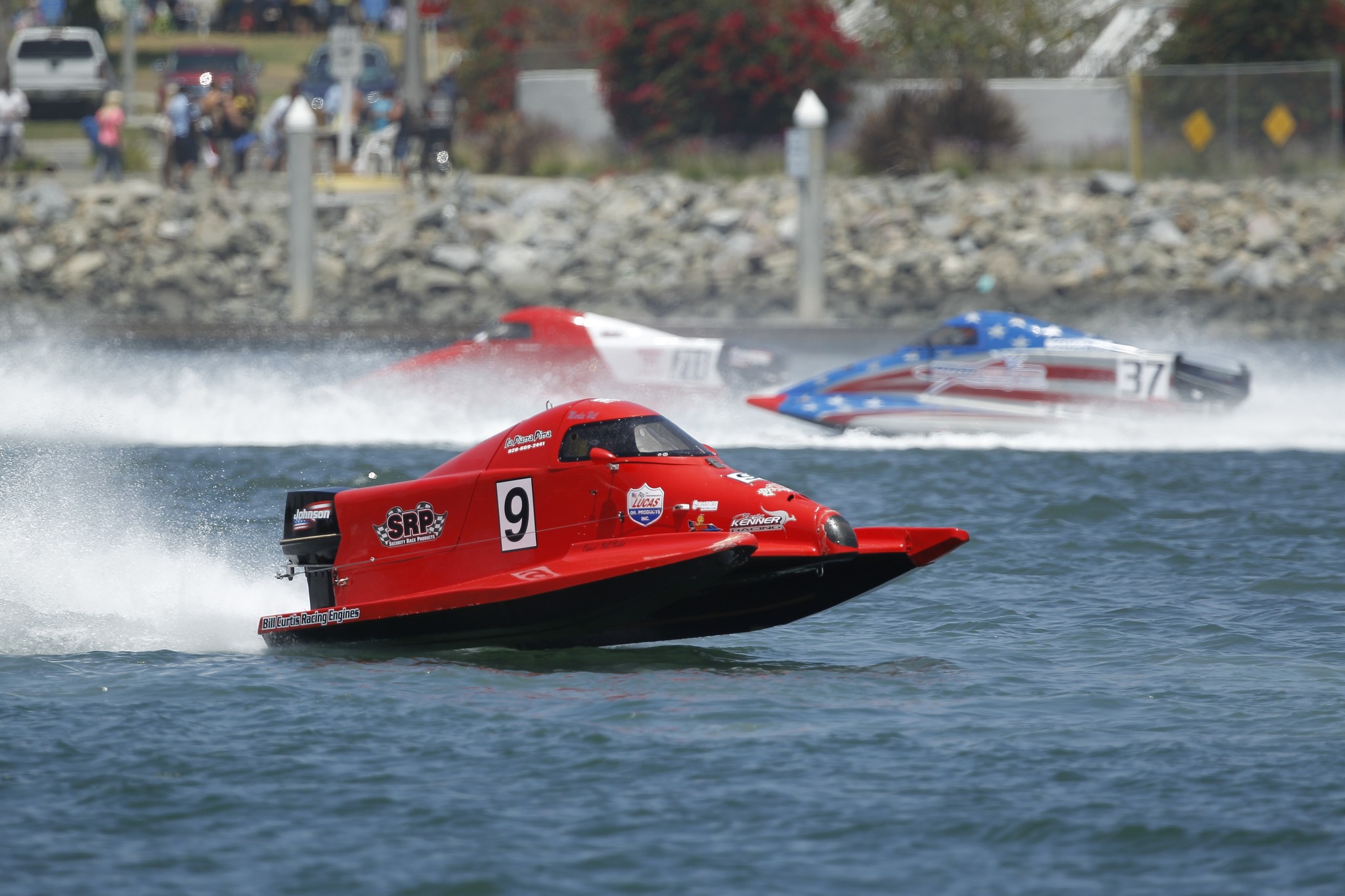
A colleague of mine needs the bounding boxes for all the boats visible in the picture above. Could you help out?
[347,302,777,407]
[259,396,970,656]
[752,306,1255,436]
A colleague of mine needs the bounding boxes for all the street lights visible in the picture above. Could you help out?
[785,85,827,324]
[282,97,316,324]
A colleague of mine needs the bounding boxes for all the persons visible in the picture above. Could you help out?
[0,69,31,190]
[95,79,405,178]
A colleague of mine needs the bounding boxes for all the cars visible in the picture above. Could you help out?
[290,40,396,115]
[160,41,251,125]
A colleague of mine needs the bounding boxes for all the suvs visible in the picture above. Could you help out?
[5,27,116,116]
